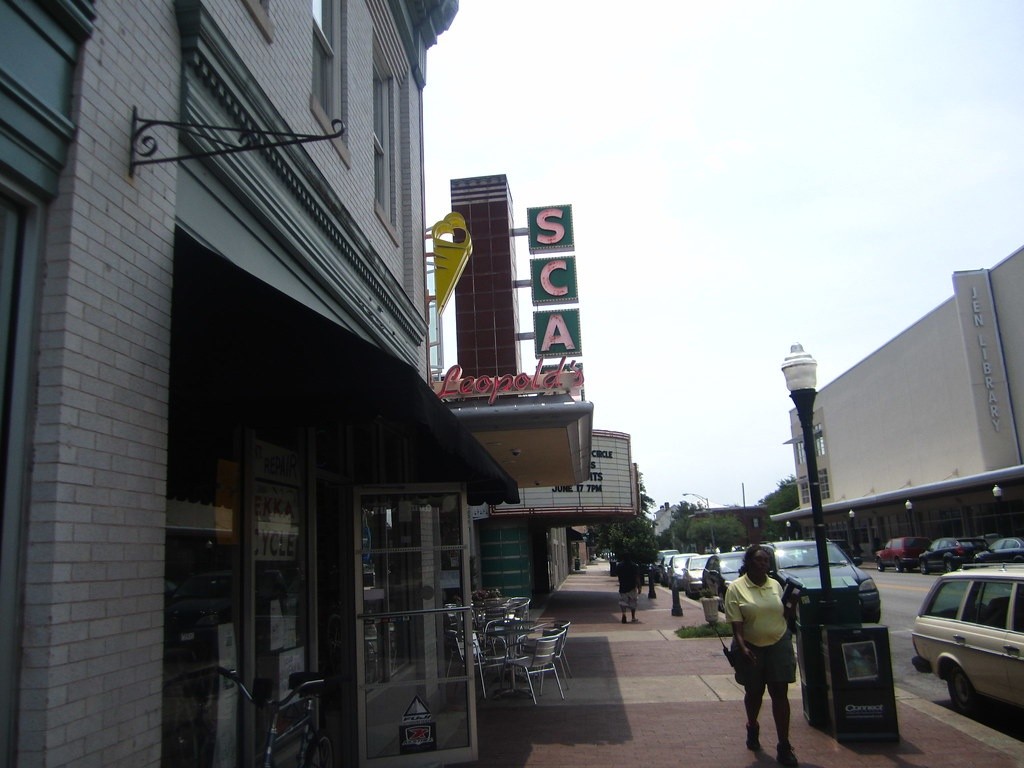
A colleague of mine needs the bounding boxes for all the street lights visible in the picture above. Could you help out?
[848,509,858,551]
[682,493,716,547]
[992,485,1004,535]
[905,499,915,537]
[781,341,841,625]
[785,520,791,540]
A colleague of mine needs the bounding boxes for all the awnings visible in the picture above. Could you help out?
[175,219,522,506]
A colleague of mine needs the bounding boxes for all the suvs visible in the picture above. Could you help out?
[917,536,990,574]
[911,563,1024,716]
[651,549,680,583]
[873,537,933,572]
[751,536,882,634]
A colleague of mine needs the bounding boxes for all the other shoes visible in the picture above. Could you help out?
[622,616,626,622]
[632,617,636,623]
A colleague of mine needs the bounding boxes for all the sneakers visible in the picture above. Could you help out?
[746,721,760,749]
[777,737,798,765]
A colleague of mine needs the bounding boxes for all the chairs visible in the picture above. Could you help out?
[444,596,573,705]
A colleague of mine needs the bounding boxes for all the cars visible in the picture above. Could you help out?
[659,555,671,587]
[162,572,242,654]
[973,537,1024,563]
[701,551,747,614]
[683,553,713,601]
[803,540,863,568]
[668,553,701,590]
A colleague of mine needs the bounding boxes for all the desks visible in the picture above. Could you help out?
[488,622,547,698]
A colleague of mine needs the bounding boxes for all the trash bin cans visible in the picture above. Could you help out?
[575,558,581,570]
[610,561,616,576]
[819,621,900,743]
[794,575,860,726]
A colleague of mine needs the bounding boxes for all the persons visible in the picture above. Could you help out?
[724,545,798,768]
[617,551,643,624]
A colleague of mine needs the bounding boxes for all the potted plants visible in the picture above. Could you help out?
[700,588,721,624]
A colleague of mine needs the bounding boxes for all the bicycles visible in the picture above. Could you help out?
[191,664,334,768]
